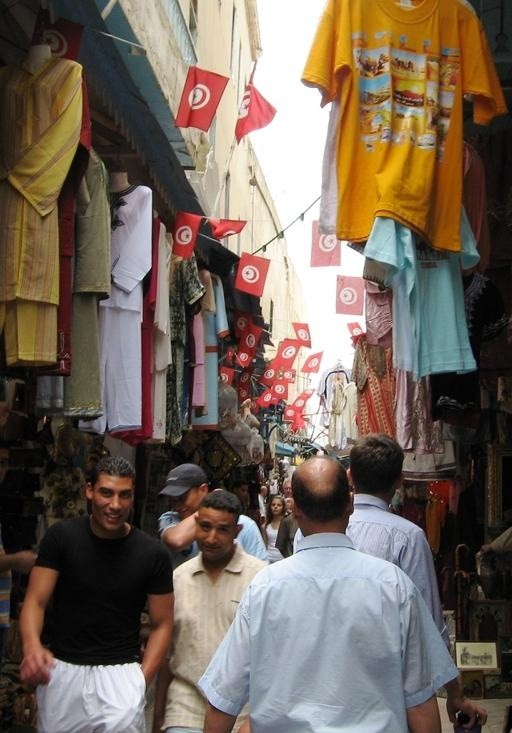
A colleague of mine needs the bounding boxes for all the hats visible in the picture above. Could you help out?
[157,459,206,498]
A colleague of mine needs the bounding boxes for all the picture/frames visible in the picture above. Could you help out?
[469,600,506,641]
[484,674,512,698]
[454,641,501,671]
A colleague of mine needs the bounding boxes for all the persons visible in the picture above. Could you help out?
[15,456,179,731]
[194,454,466,732]
[0,442,39,656]
[155,461,266,570]
[164,489,270,732]
[288,432,489,732]
[248,453,314,565]
[2,42,219,435]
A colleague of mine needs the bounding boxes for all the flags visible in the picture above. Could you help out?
[222,317,326,438]
[208,218,248,239]
[309,220,342,267]
[174,66,230,136]
[335,273,364,315]
[346,322,363,337]
[236,80,278,145]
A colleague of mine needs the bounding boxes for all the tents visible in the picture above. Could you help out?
[236,252,271,307]
